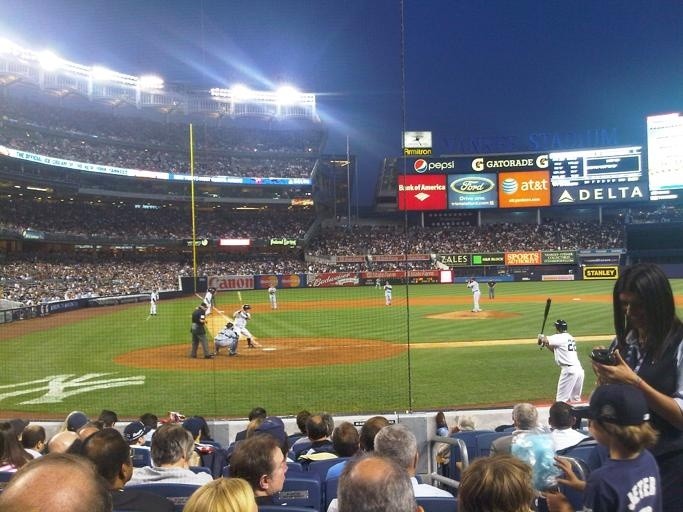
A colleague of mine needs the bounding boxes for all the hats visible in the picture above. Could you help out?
[65,410,90,431]
[182,416,205,435]
[123,421,151,442]
[570,383,651,425]
[9,418,30,436]
[254,416,285,448]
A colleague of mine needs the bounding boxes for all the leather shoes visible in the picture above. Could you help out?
[435,411,448,435]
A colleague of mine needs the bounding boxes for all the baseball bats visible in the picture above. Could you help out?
[237,291,248,318]
[538,299,552,347]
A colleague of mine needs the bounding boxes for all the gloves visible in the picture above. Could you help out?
[537,334,544,342]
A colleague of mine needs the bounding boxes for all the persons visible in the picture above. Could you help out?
[1,384,663,512]
[150,289,158,315]
[538,319,584,404]
[190,303,254,358]
[467,278,480,312]
[1,99,683,310]
[589,264,683,511]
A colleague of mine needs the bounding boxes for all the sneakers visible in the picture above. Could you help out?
[229,352,240,356]
[191,354,196,358]
[204,355,215,359]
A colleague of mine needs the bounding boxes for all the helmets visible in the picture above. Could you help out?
[242,305,251,311]
[207,287,216,294]
[552,319,567,333]
[226,323,233,328]
